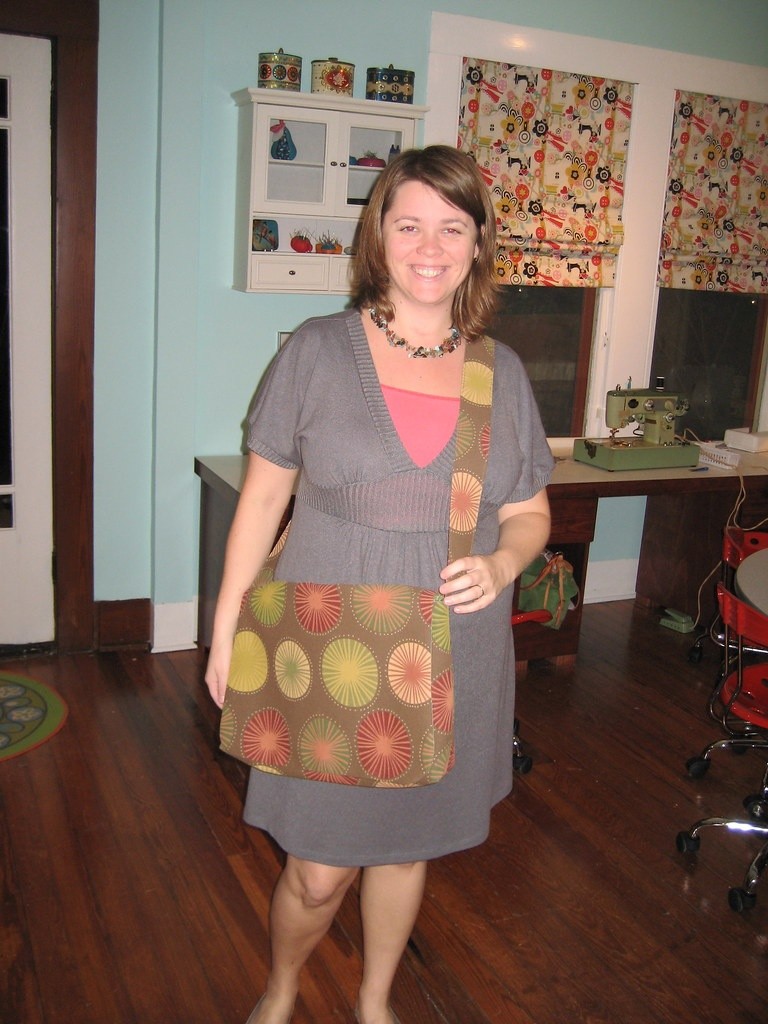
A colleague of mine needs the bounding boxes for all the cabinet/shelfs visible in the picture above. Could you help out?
[195,440,768,688]
[230,87,431,295]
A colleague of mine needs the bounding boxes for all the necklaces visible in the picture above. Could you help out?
[369,307,462,358]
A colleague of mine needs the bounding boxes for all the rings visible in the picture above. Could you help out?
[477,585,484,596]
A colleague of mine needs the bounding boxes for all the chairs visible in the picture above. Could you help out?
[677,525,768,914]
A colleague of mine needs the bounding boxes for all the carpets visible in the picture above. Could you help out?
[0,669,70,764]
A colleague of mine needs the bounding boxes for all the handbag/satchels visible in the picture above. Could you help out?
[519,550,579,630]
[218,582,457,789]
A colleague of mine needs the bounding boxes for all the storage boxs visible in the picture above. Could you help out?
[724,427,767,453]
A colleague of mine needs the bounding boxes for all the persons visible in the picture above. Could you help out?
[204,146,555,1024]
